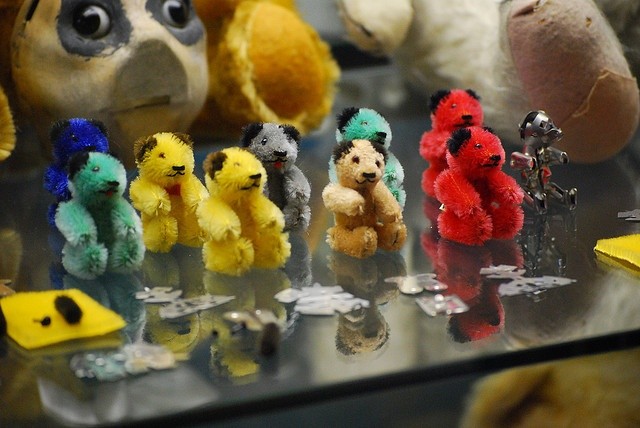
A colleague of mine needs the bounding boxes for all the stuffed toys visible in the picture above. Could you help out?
[321,140,406,259]
[54,151,145,281]
[52,234,313,386]
[419,88,485,200]
[433,126,524,246]
[418,193,524,345]
[0,0,342,164]
[238,121,312,233]
[327,105,406,214]
[324,248,409,359]
[337,0,640,167]
[130,132,209,254]
[195,147,292,278]
[46,117,109,226]
[51,233,313,387]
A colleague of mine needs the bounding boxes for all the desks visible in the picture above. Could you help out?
[0,45,639,428]
